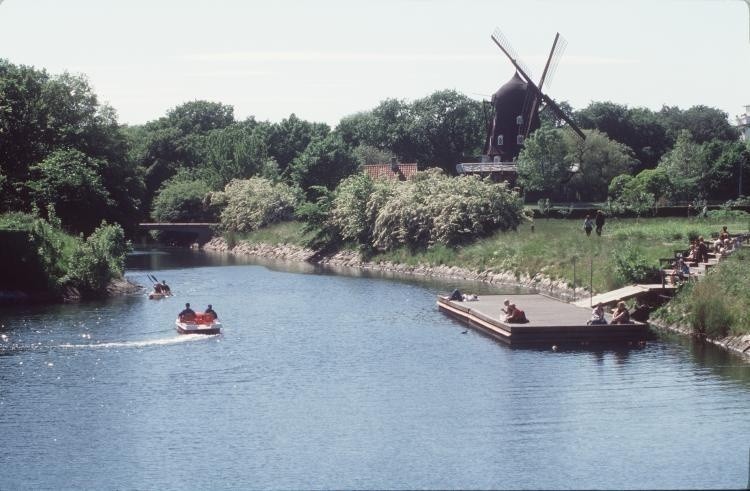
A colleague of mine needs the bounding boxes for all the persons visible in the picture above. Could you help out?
[585,302,636,325]
[595,210,605,238]
[501,300,529,324]
[530,220,535,233]
[670,225,733,286]
[449,288,480,302]
[583,215,595,239]
[178,303,218,324]
[151,279,171,294]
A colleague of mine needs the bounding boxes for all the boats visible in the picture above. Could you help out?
[149,291,171,300]
[176,311,223,335]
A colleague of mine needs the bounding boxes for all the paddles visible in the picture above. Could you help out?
[147,274,158,285]
